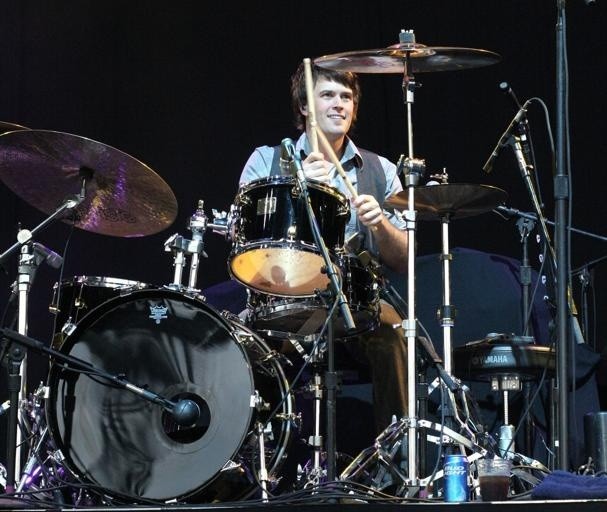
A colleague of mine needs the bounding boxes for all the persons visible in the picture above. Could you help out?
[228,62,408,492]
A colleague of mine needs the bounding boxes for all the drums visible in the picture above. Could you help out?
[242,255,381,342]
[227,175,351,298]
[44,289,293,501]
[56,276,158,330]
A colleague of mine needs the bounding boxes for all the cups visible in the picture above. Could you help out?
[477,458,512,502]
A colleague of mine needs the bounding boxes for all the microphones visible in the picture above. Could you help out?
[173,400,202,426]
[31,242,64,270]
[279,137,297,177]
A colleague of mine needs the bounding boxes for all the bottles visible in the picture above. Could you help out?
[583,410,606,477]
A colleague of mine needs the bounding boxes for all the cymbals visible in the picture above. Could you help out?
[383,183,508,222]
[0,131,178,238]
[313,47,501,74]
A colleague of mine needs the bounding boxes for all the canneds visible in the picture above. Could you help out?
[443,454,469,502]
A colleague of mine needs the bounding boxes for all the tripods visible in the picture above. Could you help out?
[336,101,544,505]
[351,220,550,493]
